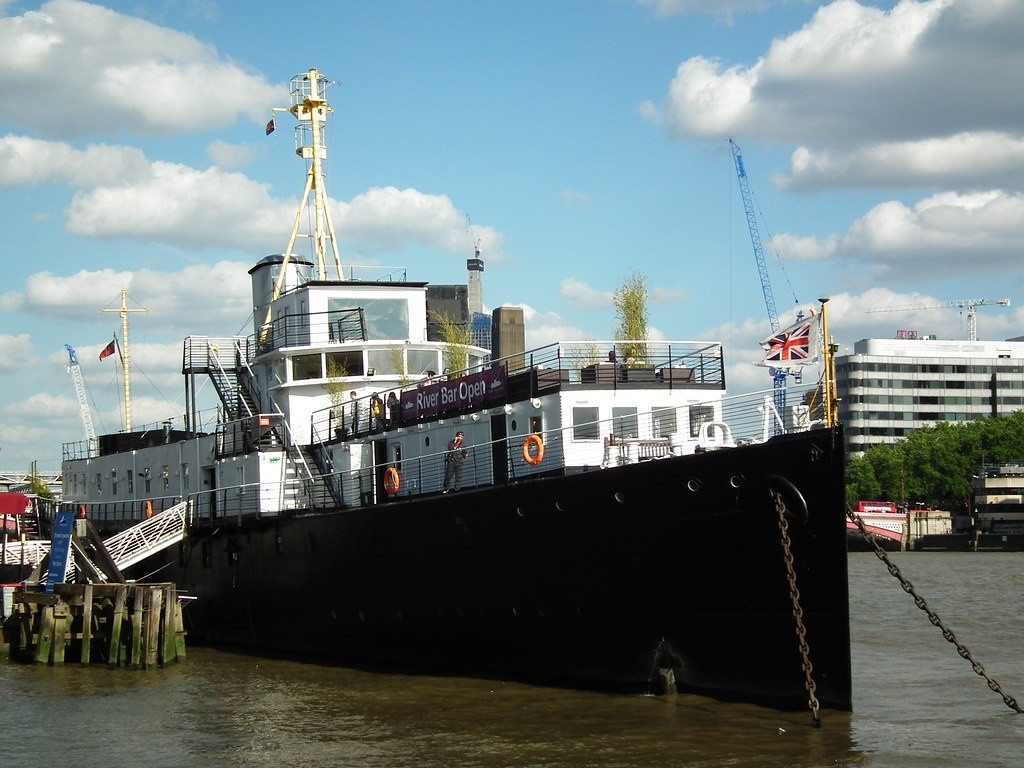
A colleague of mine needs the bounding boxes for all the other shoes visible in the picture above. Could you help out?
[443,489,449,493]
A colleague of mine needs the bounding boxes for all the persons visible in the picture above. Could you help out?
[417,370,436,388]
[442,431,468,494]
[605,351,618,364]
[350,390,400,436]
[622,352,638,369]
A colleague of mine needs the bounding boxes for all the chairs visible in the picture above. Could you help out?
[537,369,568,390]
[581,364,695,384]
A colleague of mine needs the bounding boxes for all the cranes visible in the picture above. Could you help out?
[864,294,1013,339]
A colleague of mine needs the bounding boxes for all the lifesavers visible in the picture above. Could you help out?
[383,468,399,493]
[523,435,544,466]
[145,501,152,519]
[77,504,86,518]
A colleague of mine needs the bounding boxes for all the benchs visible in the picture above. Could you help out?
[637,443,683,462]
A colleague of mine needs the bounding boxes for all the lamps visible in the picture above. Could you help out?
[443,368,450,374]
[427,371,436,377]
[367,368,375,375]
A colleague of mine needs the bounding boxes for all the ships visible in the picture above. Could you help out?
[51,66,857,720]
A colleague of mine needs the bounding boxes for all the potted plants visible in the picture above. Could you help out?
[324,355,351,439]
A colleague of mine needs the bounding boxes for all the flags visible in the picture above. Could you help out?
[753,313,821,366]
[265,119,274,136]
